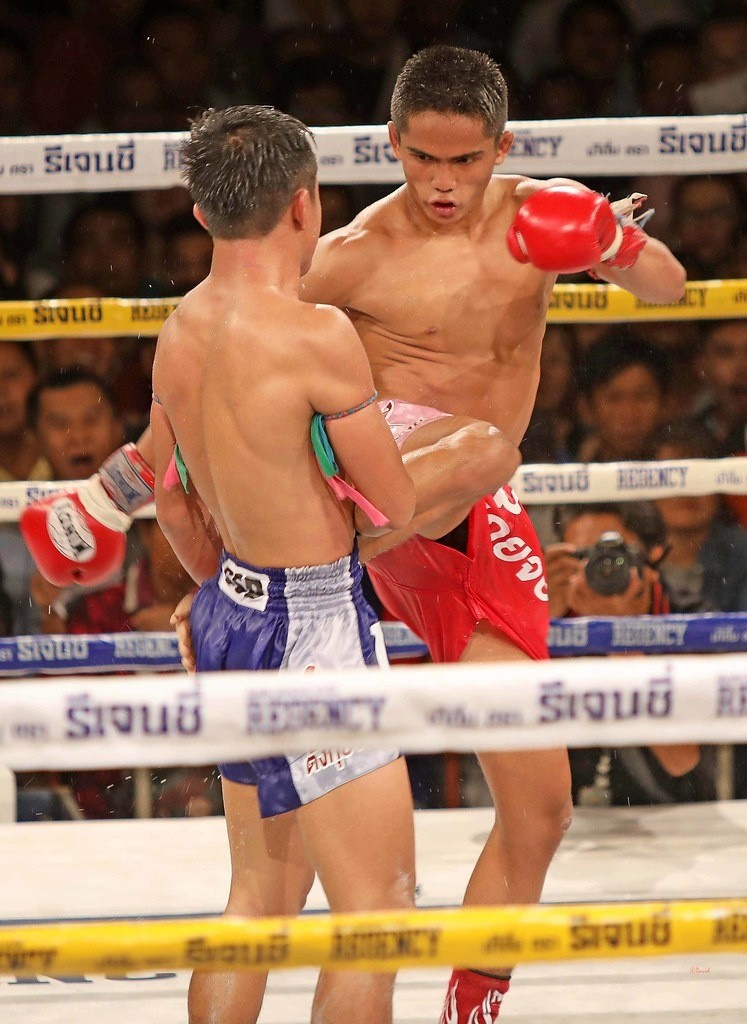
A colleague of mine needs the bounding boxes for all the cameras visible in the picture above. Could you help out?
[568,532,647,596]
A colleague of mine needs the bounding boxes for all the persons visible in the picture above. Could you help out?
[18,41,687,1023]
[153,105,414,1023]
[2,1,747,824]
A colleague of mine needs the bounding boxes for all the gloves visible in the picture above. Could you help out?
[507,184,647,280]
[19,442,156,587]
